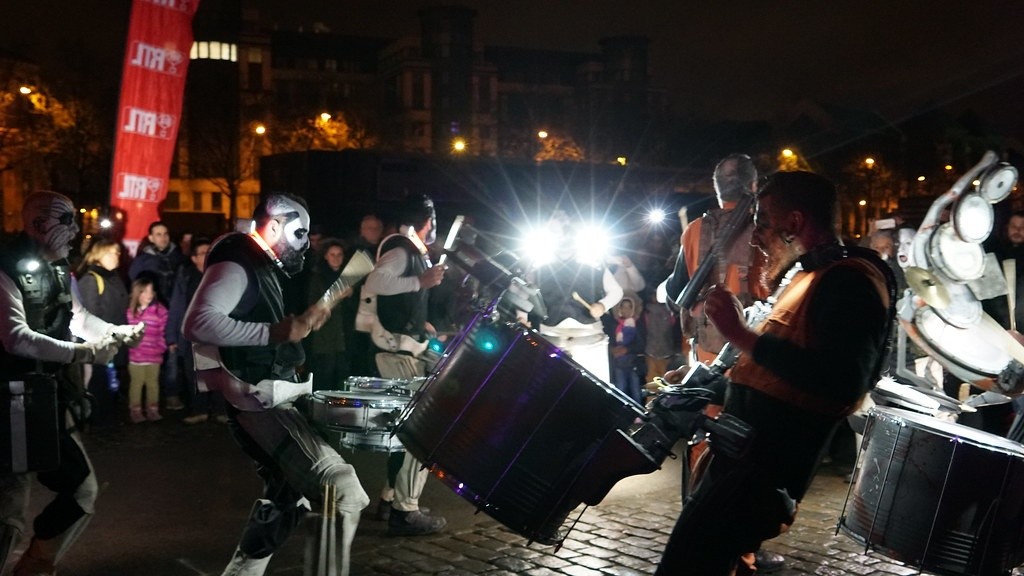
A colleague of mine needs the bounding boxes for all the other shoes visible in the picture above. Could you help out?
[845,469,859,482]
[166,396,184,410]
[145,403,163,421]
[13,550,56,576]
[215,415,231,422]
[129,406,146,423]
[183,412,208,423]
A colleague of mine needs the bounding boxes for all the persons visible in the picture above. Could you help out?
[665,154,784,576]
[654,168,898,576]
[0,189,146,576]
[355,192,448,531]
[181,192,370,576]
[63,209,1024,484]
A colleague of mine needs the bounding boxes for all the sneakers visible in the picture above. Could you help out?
[377,500,430,519]
[388,511,447,536]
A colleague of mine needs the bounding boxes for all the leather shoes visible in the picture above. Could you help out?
[754,548,785,573]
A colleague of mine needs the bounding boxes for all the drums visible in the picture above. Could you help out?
[834,380,1024,576]
[310,375,434,454]
[441,224,524,294]
[392,303,645,546]
[913,160,1024,378]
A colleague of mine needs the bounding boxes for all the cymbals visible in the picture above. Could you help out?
[904,266,952,310]
